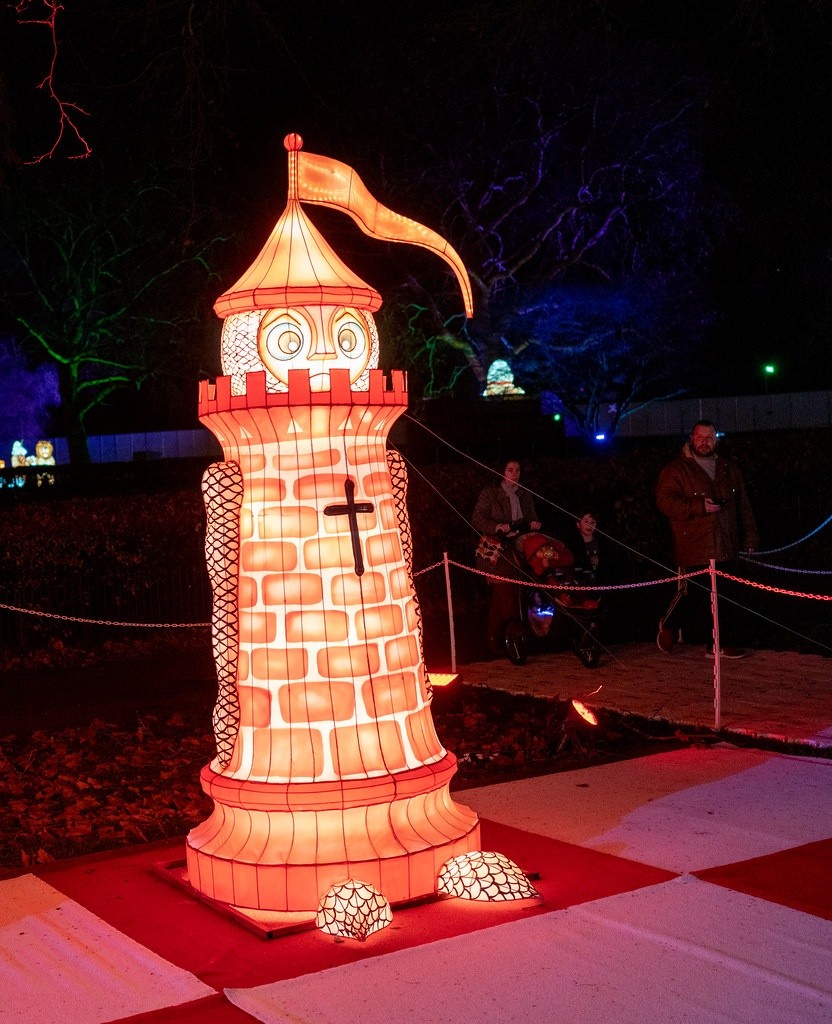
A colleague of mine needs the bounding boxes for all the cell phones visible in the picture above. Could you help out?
[713,498,729,507]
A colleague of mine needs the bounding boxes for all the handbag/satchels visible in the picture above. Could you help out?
[474,536,506,565]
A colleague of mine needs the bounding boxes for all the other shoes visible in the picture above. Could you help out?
[486,641,506,656]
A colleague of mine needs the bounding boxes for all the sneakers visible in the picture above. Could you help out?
[704,645,746,659]
[658,621,672,653]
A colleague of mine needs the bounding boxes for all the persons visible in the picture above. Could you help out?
[574,509,602,654]
[657,419,758,658]
[536,547,597,608]
[472,451,542,665]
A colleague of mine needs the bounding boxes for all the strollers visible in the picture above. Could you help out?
[498,523,610,669]
